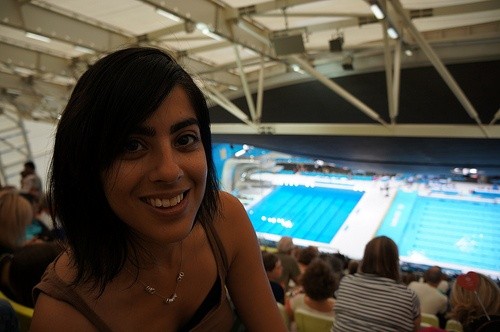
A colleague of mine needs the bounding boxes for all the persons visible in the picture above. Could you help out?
[262,236,500,332]
[0,160,70,332]
[28,44,290,332]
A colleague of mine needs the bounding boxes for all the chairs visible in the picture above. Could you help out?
[0,244,468,332]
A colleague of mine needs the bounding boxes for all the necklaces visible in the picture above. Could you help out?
[122,238,184,304]
